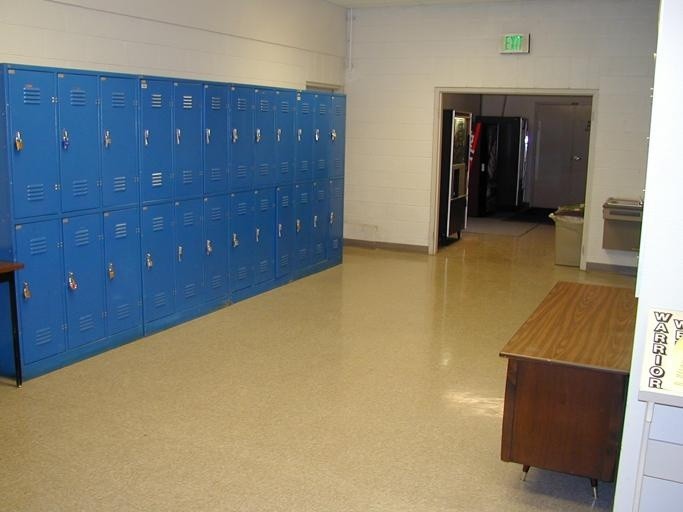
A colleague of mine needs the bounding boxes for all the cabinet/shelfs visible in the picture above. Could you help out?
[472,116,527,216]
[0,64,347,383]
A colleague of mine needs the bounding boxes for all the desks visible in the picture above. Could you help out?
[0,257,25,391]
[495,278,637,501]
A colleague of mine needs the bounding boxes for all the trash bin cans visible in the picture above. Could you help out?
[549,203,584,267]
